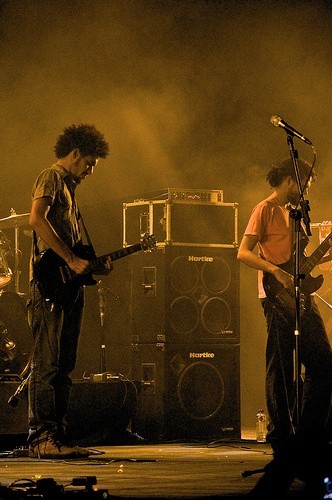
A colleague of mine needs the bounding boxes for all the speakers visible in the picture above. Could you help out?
[129,246,241,343]
[129,343,241,443]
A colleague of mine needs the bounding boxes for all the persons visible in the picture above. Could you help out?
[236,158,332,453]
[27,125,114,459]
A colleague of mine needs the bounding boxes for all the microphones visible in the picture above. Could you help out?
[270,115,313,146]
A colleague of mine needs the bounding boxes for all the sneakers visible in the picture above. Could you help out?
[26,427,76,458]
[50,429,89,457]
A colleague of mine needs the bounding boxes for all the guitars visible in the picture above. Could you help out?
[33,231,160,309]
[262,230,331,326]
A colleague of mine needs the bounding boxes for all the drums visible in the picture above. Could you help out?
[0,289,36,381]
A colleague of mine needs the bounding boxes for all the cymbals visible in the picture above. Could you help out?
[0,213,32,230]
